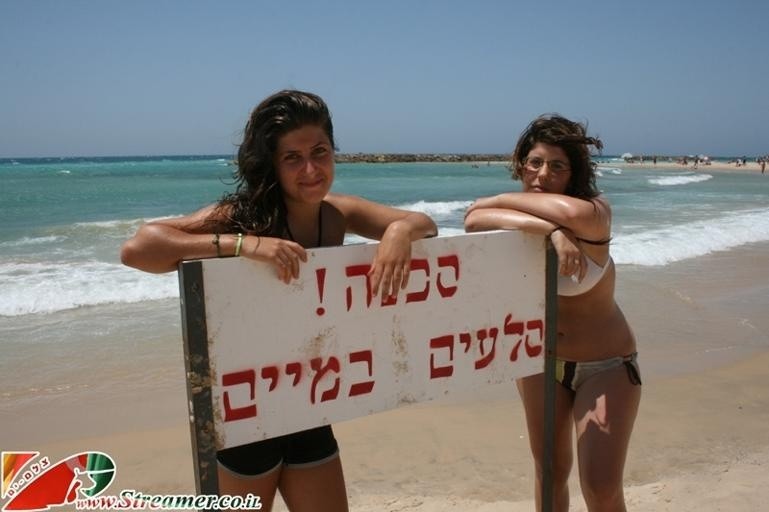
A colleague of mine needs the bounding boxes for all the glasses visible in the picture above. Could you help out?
[524,157,572,171]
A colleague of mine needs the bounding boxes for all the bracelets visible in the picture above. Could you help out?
[547,225,569,243]
[232,231,247,257]
[213,233,222,259]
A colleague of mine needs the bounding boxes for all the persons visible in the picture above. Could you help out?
[119,88,438,512]
[463,110,643,512]
[593,150,769,175]
